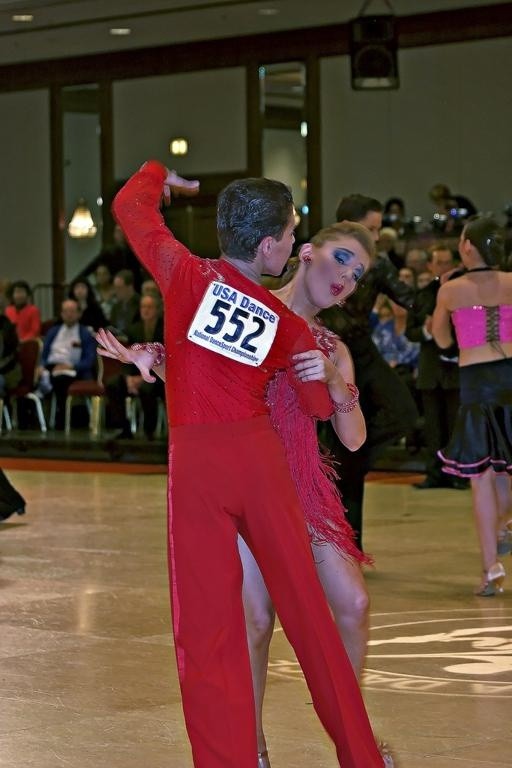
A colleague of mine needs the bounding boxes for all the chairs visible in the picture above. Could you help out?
[1,355,173,447]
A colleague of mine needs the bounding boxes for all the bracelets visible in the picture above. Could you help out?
[329,383,361,413]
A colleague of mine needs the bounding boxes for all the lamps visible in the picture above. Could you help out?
[348,1,402,93]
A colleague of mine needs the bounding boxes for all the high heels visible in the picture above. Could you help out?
[496,519,512,556]
[473,562,506,596]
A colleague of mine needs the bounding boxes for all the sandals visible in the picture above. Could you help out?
[257,750,270,768]
[376,740,394,768]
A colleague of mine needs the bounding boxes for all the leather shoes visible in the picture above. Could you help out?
[446,479,471,489]
[412,475,443,489]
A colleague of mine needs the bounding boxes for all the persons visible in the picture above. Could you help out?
[111,161,386,768]
[429,217,510,596]
[235,221,398,767]
[2,185,512,574]
